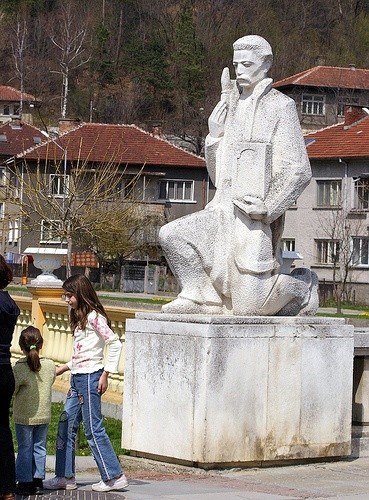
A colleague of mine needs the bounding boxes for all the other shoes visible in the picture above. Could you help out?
[14,482,31,495]
[0,490,16,500]
[30,479,43,494]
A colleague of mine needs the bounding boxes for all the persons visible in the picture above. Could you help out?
[0,253,20,500]
[156,34,321,321]
[40,274,131,492]
[11,325,57,496]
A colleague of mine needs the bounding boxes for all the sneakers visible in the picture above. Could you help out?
[42,475,77,490]
[91,474,129,492]
